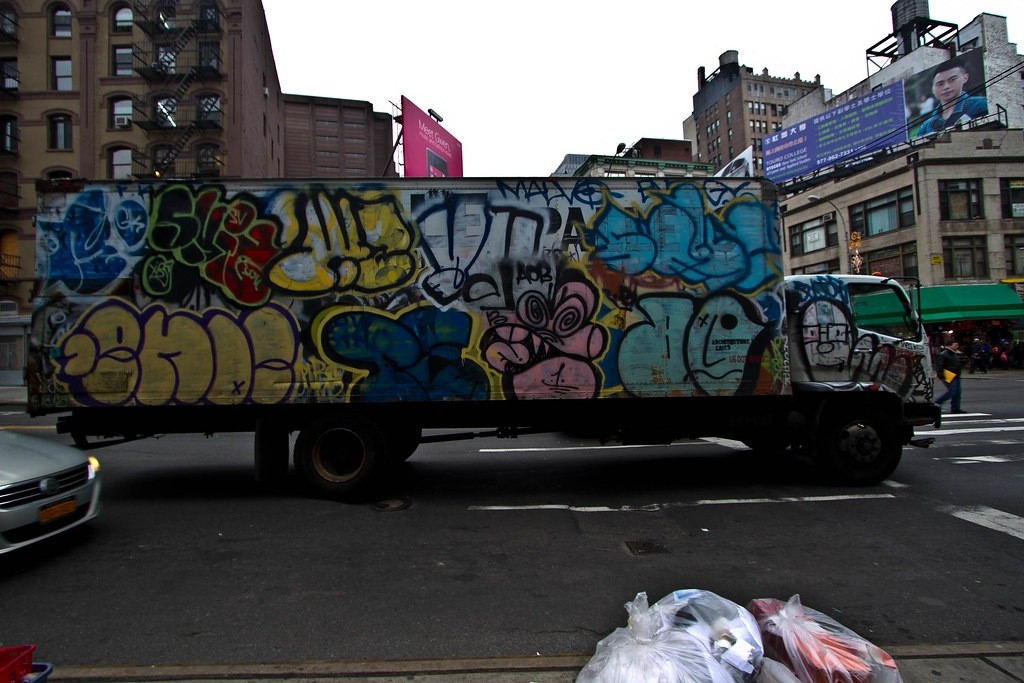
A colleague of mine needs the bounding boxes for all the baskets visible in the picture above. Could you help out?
[0,644,36,683]
[22,662,53,683]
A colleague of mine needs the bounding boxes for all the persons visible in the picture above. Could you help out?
[906,59,988,139]
[965,336,1017,374]
[933,339,967,415]
[935,345,945,370]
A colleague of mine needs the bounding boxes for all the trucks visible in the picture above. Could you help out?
[23,175,944,503]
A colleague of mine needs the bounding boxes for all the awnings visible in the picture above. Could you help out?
[848,281,1024,327]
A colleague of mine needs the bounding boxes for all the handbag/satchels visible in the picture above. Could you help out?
[574,588,900,682]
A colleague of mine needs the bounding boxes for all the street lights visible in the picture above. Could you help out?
[807,195,852,274]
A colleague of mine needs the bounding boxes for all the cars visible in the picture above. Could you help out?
[0,428,104,557]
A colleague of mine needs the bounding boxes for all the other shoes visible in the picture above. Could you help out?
[951,408,968,414]
